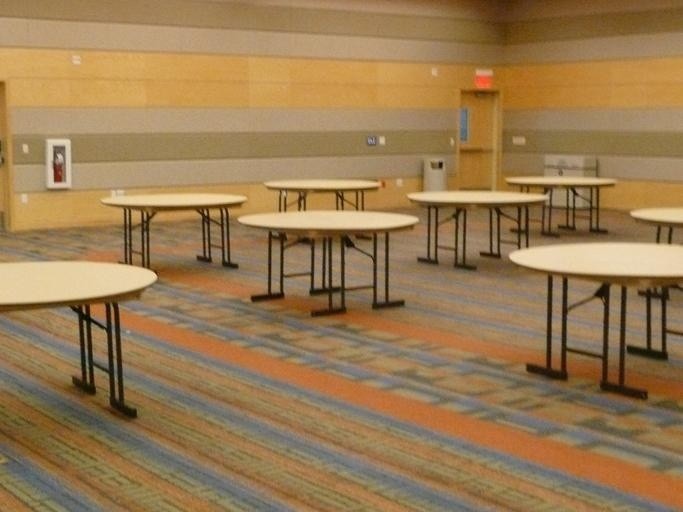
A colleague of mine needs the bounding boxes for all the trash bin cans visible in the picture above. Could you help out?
[424,159,445,191]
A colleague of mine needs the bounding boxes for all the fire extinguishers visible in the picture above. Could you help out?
[55,149,63,182]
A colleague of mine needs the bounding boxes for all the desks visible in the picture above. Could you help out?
[505,176,617,237]
[263,179,380,244]
[509,242,683,400]
[237,209,419,317]
[630,207,683,300]
[101,193,247,273]
[0,261,158,418]
[407,192,549,269]
